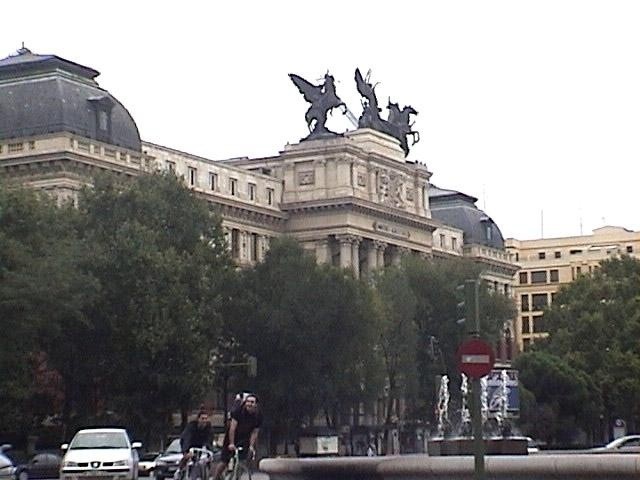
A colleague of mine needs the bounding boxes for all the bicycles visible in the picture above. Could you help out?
[182,447,216,480]
[220,448,256,480]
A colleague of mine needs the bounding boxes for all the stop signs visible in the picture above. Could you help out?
[457,341,494,378]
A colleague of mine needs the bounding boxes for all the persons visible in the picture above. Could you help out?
[173,410,214,479]
[211,392,266,480]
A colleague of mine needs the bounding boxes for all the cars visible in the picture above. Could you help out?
[0,443,17,480]
[588,434,640,453]
[511,435,540,454]
[15,450,63,480]
[155,438,223,480]
[139,452,158,475]
[61,427,143,480]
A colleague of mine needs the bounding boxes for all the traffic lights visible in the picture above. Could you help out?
[457,284,466,329]
